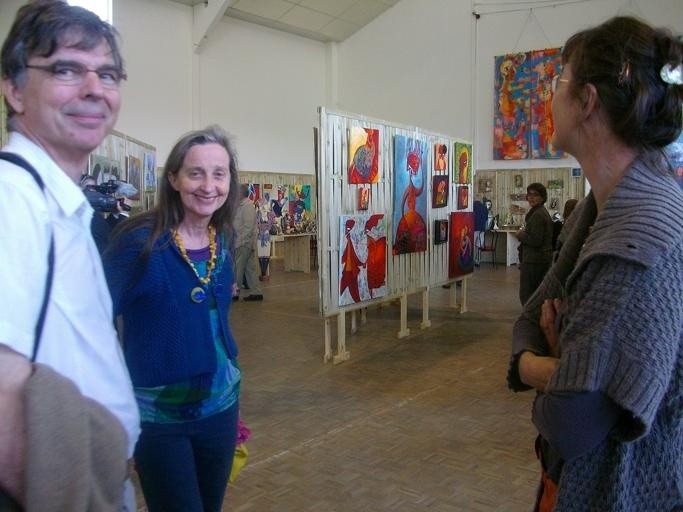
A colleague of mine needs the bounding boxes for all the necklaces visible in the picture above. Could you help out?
[164,221,221,305]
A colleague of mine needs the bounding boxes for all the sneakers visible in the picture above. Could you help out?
[243,295,263,301]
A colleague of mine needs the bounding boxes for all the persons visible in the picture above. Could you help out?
[228,183,264,303]
[99,126,244,511]
[79,175,133,257]
[513,182,553,306]
[472,191,488,266]
[504,11,682,511]
[0,0,142,511]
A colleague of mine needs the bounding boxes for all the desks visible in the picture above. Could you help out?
[491,228,524,267]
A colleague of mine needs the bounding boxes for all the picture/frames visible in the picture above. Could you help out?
[435,219,448,244]
[458,186,468,209]
[127,155,141,200]
[433,175,449,208]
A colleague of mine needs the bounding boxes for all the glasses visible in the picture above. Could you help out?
[26,60,127,85]
[551,75,569,93]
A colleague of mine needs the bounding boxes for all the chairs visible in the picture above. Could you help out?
[478,227,500,269]
